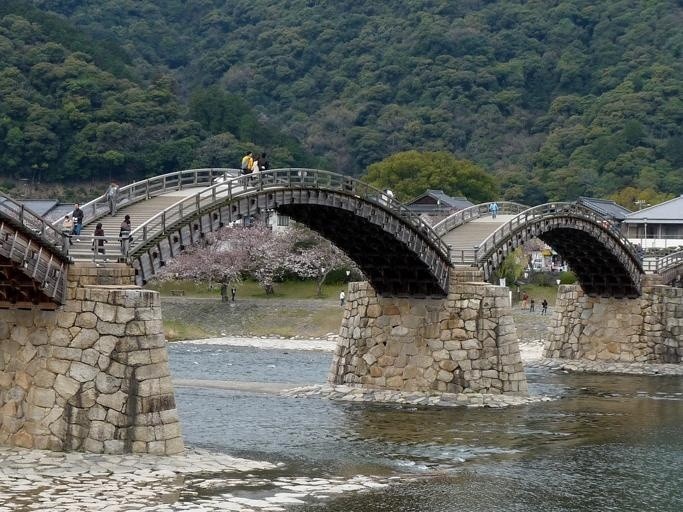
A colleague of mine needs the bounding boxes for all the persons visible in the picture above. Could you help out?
[90,222,108,263]
[256,152,268,192]
[118,215,132,247]
[230,284,236,301]
[241,150,254,188]
[489,200,497,220]
[380,186,395,204]
[61,216,74,244]
[71,203,84,242]
[339,289,346,306]
[541,299,547,316]
[251,155,259,177]
[529,297,535,313]
[104,180,119,217]
[344,175,353,190]
[519,290,530,310]
[634,242,643,263]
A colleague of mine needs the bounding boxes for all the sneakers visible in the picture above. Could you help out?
[76,239,80,242]
[104,259,109,262]
[112,215,115,217]
[108,212,111,215]
